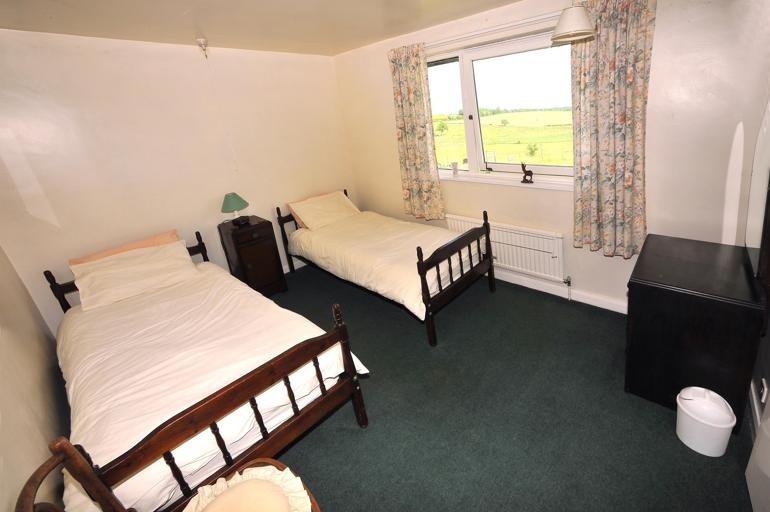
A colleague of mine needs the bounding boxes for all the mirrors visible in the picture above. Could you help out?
[745,99,770,276]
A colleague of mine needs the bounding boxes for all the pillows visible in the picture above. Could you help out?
[289,191,359,231]
[286,193,330,228]
[70,227,178,265]
[68,240,203,311]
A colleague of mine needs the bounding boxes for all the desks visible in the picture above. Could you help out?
[623,233,766,436]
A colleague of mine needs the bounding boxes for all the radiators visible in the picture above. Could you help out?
[445,214,571,300]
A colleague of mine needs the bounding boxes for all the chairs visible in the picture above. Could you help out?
[15,437,321,512]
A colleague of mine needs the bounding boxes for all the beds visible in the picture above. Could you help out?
[275,189,496,347]
[42,232,372,512]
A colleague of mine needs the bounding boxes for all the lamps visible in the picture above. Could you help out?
[221,192,249,218]
[551,0,595,41]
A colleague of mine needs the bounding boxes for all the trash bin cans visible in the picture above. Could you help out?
[676,386,737,457]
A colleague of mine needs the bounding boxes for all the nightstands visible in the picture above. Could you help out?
[218,215,288,298]
